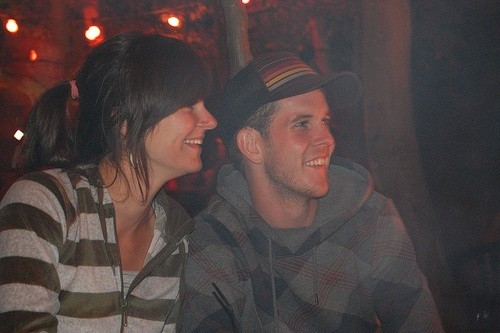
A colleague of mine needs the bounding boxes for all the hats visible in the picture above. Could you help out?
[215,51,364,138]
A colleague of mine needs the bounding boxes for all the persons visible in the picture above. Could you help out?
[182,47,449,332]
[0,30,216,333]
